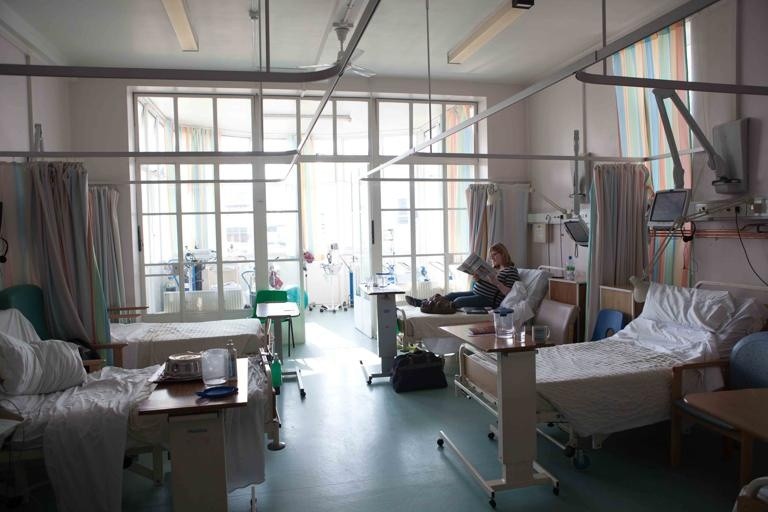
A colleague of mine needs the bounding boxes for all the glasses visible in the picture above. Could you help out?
[490,252,498,259]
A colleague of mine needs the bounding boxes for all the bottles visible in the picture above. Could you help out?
[225,340,237,381]
[565,256,575,281]
[271,353,282,387]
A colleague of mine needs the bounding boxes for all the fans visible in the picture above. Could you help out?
[298,20,377,80]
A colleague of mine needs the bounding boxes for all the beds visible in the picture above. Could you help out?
[399,266,554,352]
[453,280,767,471]
[1,309,268,512]
[106,320,271,369]
[731,477,767,511]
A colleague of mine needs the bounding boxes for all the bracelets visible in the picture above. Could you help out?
[495,281,502,288]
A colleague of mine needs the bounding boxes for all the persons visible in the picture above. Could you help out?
[405,243,519,309]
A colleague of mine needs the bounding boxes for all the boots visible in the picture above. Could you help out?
[406,296,422,307]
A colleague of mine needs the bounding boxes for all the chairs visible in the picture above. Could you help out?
[1,284,130,371]
[536,300,580,346]
[251,290,297,360]
[667,326,767,483]
[587,308,626,343]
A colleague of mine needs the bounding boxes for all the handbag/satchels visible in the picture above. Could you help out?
[389,350,448,393]
[420,294,456,314]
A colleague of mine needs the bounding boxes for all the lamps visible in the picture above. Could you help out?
[443,0,535,69]
[158,1,202,53]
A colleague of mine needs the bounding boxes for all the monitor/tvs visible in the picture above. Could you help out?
[646,189,691,230]
[562,218,589,246]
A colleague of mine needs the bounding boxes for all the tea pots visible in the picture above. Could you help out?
[493,306,514,338]
[201,348,228,385]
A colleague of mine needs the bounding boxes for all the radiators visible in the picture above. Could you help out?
[164,288,242,311]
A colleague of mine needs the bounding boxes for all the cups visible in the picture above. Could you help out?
[515,325,526,343]
[532,325,550,343]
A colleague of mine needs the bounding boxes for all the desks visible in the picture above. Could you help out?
[436,321,562,508]
[255,301,301,355]
[358,280,406,385]
[684,386,767,443]
[135,355,249,512]
